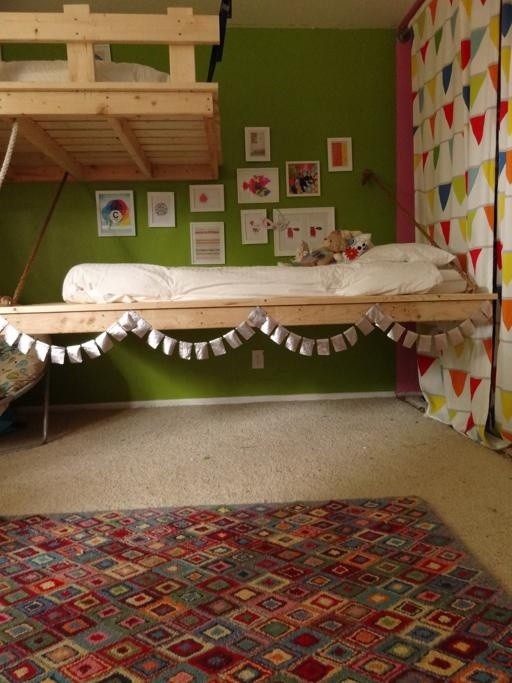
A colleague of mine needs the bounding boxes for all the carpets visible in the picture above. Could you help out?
[0,496,512,683]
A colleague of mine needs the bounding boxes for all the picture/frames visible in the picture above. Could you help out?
[96,126,353,265]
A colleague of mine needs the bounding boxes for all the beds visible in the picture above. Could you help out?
[0,247,499,445]
[0,0,221,180]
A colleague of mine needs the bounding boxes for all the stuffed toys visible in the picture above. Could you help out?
[277,228,347,267]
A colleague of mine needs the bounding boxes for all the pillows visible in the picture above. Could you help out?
[355,242,457,267]
